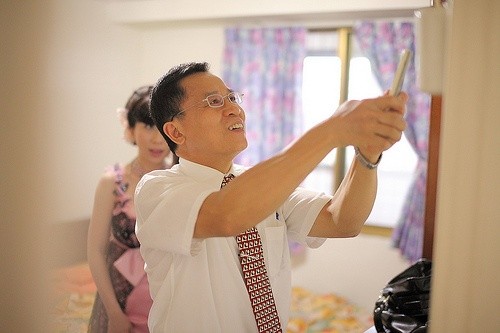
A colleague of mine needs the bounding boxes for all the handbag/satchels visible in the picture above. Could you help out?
[374,257,432,333]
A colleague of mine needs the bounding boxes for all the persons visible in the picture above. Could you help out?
[89,85,181,333]
[133,62,408,333]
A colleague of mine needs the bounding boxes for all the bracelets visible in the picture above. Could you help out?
[352,145,383,169]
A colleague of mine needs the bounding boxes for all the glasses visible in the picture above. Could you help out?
[170,91,244,122]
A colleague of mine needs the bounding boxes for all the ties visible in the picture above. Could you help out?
[221,173,283,333]
